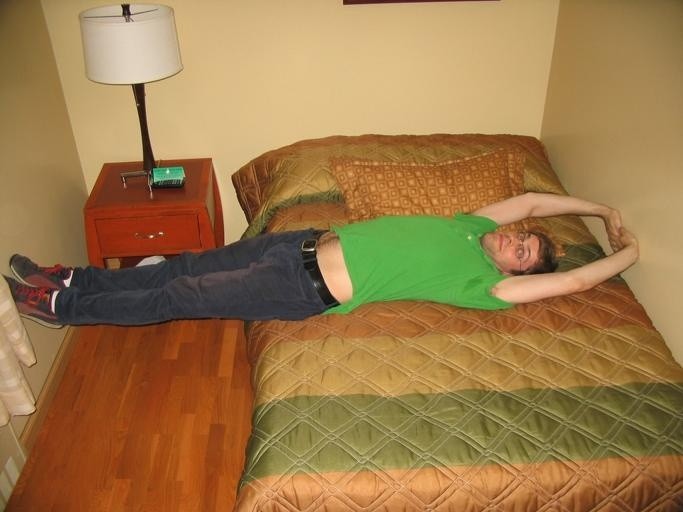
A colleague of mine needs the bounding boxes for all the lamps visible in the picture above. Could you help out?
[77,1,185,199]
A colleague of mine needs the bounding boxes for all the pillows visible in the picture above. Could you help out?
[330,147,526,224]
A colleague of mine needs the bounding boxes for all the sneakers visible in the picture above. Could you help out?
[2,254,73,329]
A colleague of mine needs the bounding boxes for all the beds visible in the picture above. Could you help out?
[232,132,683,511]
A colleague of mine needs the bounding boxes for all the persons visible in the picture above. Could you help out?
[3,192,639,329]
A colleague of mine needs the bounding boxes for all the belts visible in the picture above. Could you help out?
[301,230,340,309]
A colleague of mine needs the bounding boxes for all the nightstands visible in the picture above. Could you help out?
[84,158,227,270]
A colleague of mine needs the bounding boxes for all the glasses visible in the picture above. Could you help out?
[516,232,525,272]
